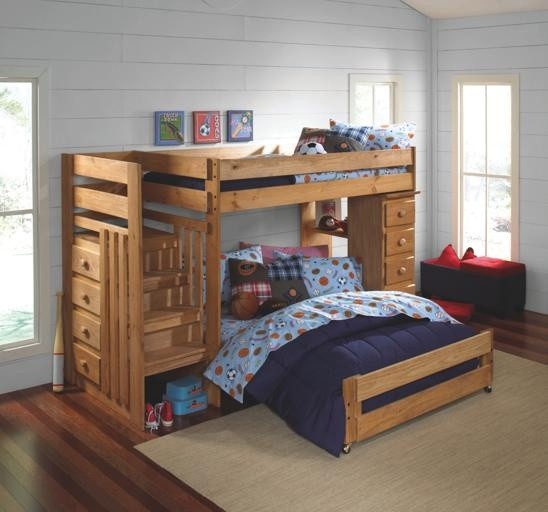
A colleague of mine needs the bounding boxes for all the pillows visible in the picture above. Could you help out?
[273,250,364,297]
[228,255,309,320]
[435,244,477,268]
[293,119,418,170]
[239,241,329,264]
[181,246,263,306]
[432,296,475,323]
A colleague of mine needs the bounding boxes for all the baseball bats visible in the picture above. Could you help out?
[52,291,64,392]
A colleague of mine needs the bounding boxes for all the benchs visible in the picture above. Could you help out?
[420,256,526,319]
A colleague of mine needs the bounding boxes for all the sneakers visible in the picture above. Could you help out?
[146,403,158,429]
[159,401,175,427]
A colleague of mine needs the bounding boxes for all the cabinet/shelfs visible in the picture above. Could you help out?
[70,223,192,419]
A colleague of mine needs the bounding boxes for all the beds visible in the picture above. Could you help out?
[137,146,415,213]
[201,299,494,452]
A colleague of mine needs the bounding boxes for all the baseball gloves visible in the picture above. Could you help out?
[319,214,342,231]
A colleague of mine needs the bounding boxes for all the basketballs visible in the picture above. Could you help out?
[231,292,258,320]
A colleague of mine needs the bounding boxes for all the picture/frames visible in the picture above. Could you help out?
[154,110,253,147]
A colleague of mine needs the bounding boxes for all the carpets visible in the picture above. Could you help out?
[133,348,548,511]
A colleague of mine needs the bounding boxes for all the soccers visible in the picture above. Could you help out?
[300,142,326,155]
[200,124,210,136]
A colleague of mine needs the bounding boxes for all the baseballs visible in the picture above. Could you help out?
[325,218,335,227]
